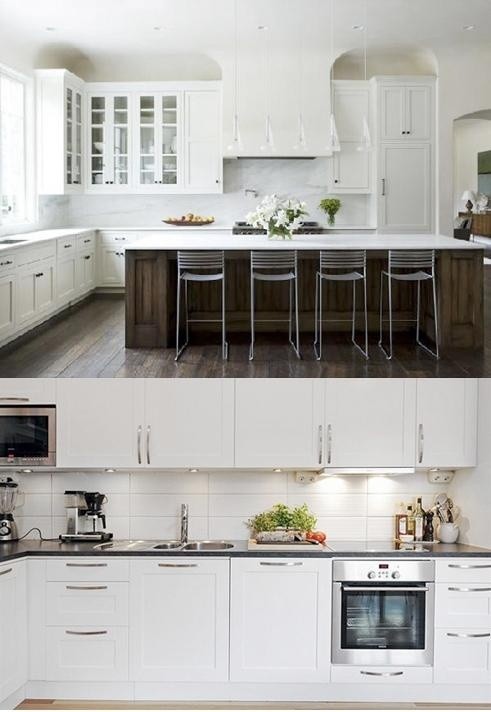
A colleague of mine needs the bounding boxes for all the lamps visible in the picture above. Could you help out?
[318,467,414,478]
[459,189,476,213]
[226,0,374,154]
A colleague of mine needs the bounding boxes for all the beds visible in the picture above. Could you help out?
[122,233,488,354]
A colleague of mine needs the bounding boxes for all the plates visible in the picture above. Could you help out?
[161,220,215,226]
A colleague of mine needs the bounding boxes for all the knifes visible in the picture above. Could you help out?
[305,539,319,544]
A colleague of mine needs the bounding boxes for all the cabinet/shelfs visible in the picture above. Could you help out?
[97,230,136,295]
[0,557,27,710]
[328,79,372,194]
[1,229,96,347]
[34,69,222,194]
[369,77,435,232]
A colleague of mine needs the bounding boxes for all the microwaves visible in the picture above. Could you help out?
[0,405,56,466]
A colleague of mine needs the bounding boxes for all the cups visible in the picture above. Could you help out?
[164,144,171,154]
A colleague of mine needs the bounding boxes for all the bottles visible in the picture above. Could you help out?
[94,174,103,184]
[395,496,460,542]
[171,136,177,153]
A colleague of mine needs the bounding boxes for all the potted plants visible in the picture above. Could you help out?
[316,198,343,227]
[240,503,319,544]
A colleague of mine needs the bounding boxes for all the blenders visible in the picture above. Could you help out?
[0,481,20,545]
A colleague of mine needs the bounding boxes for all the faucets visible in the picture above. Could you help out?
[180,502,189,542]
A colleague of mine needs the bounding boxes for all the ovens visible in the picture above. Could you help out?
[331,580,436,664]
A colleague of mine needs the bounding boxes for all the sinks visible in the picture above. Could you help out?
[182,540,234,550]
[154,543,182,548]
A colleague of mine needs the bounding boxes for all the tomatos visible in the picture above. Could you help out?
[306,531,326,544]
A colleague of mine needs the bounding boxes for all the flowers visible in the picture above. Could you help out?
[245,193,311,242]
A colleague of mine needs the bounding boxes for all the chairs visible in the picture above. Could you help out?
[453,217,473,242]
[173,248,439,362]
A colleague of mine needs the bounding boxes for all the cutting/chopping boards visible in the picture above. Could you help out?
[249,538,323,551]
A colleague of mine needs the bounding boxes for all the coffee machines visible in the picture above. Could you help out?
[58,491,115,542]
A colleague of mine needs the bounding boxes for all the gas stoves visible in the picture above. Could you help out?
[238,156,318,160]
[233,221,322,235]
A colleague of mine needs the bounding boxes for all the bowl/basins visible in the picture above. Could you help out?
[141,118,154,123]
[93,142,103,154]
